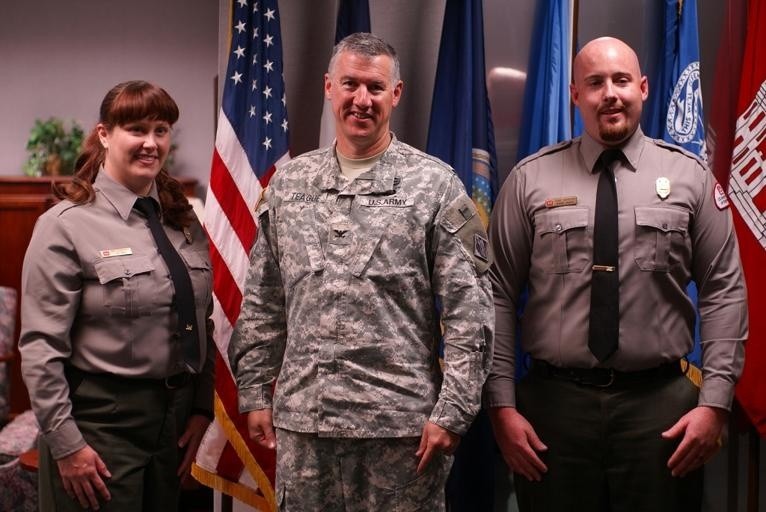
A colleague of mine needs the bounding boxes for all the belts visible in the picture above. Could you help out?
[148,372,186,388]
[530,357,687,390]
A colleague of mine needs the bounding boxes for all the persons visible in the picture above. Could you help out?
[17,76,217,508]
[487,33,749,508]
[228,29,495,512]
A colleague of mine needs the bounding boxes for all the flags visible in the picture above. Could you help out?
[189,1,292,512]
[726,1,764,446]
[644,1,715,393]
[515,0,583,392]
[314,2,373,158]
[422,0,517,400]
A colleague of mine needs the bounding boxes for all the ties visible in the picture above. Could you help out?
[587,149,620,362]
[135,197,200,372]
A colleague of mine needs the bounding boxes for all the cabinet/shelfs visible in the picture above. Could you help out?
[0,171,199,419]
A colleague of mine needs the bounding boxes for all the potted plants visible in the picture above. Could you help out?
[20,108,91,177]
